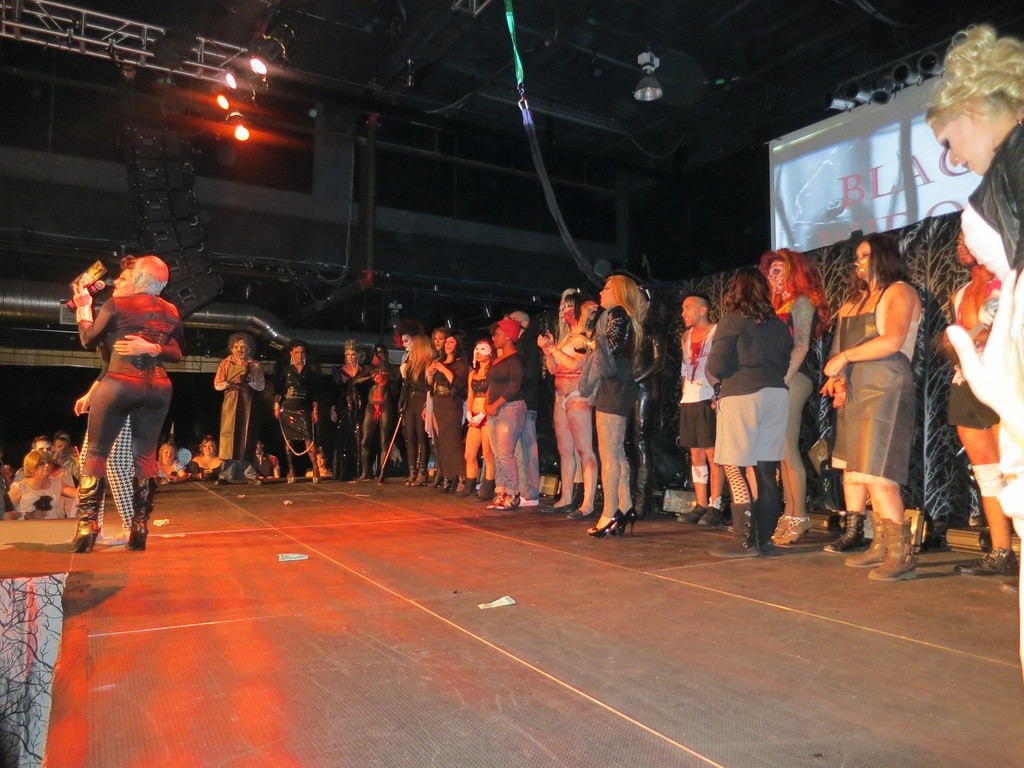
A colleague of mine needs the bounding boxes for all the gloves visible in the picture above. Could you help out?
[467,412,486,426]
[466,411,473,427]
[230,383,252,392]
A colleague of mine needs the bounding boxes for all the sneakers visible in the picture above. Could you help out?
[699,504,727,526]
[954,547,1019,575]
[677,504,707,523]
[520,496,540,507]
[496,492,520,510]
[486,492,507,509]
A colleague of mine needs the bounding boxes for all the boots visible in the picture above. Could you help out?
[127,477,156,550]
[67,477,105,554]
[709,503,758,557]
[868,518,917,581]
[823,511,865,553]
[755,498,780,557]
[845,518,888,568]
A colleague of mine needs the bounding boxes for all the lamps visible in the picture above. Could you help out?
[225,107,251,141]
[225,59,256,89]
[632,49,664,101]
[869,76,893,104]
[216,85,245,110]
[249,16,300,74]
[890,63,923,84]
[587,55,602,77]
[917,53,944,77]
[846,78,872,104]
[823,89,856,111]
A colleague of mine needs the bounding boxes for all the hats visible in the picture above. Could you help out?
[498,319,521,343]
[605,270,645,284]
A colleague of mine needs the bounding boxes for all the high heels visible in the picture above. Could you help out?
[587,509,625,539]
[774,515,811,548]
[610,508,637,535]
[771,514,795,541]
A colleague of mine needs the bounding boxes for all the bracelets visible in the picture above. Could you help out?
[844,351,850,362]
[583,338,593,349]
[152,344,160,357]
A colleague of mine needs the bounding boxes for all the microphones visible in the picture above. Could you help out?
[67,280,105,310]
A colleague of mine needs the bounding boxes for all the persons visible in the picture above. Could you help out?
[274,234,1024,595]
[154,421,223,485]
[925,26,1024,283]
[256,441,280,478]
[0,431,80,520]
[67,255,182,553]
[213,333,265,485]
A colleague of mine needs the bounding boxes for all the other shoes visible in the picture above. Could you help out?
[541,501,578,515]
[567,509,596,521]
[200,464,496,503]
